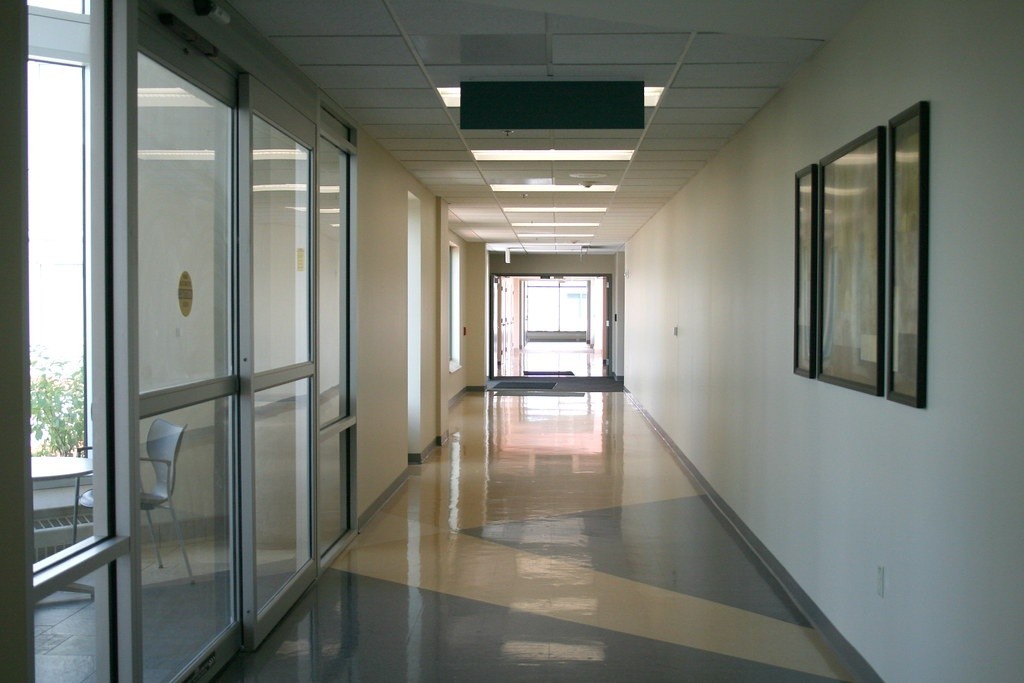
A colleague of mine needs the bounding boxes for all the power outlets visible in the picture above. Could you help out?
[876,566,885,598]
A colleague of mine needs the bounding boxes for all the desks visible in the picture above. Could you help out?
[31,456,95,600]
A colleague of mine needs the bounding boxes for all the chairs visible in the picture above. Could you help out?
[72,418,195,586]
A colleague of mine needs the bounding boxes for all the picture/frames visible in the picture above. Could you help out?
[792,163,817,379]
[886,101,927,410]
[815,125,886,397]
